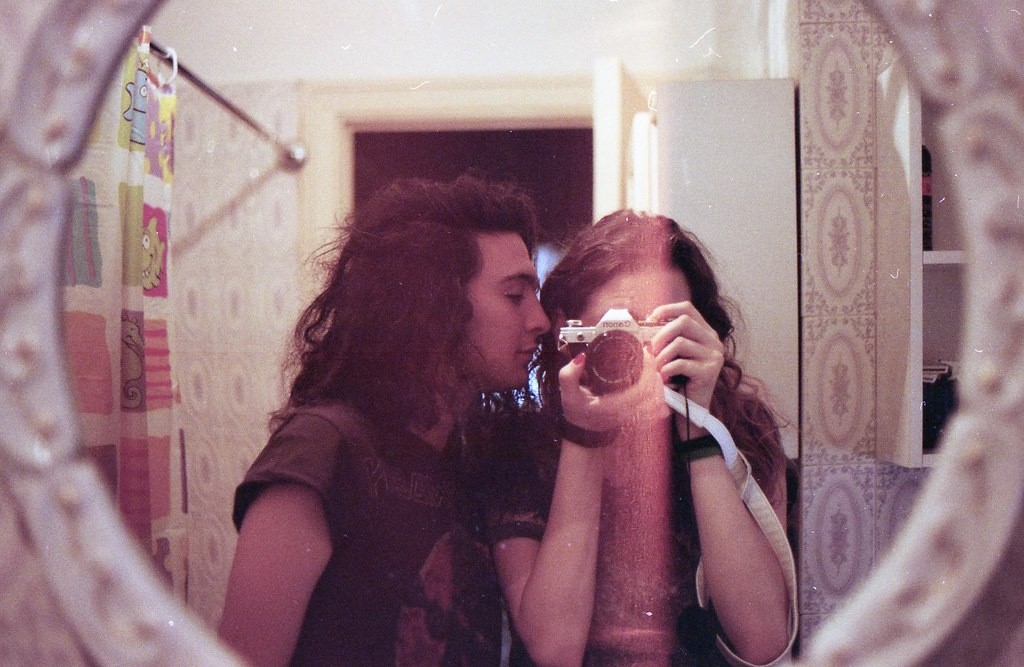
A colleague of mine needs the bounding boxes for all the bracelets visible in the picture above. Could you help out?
[679,435,723,465]
[557,420,619,449]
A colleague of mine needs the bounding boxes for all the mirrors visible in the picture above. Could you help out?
[0,0,1023,667]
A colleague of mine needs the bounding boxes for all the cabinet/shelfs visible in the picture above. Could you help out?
[876,51,968,470]
[626,79,801,462]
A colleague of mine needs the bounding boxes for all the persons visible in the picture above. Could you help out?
[214,178,551,667]
[487,209,792,667]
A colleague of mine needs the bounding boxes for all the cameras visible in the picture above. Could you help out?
[554,308,672,394]
